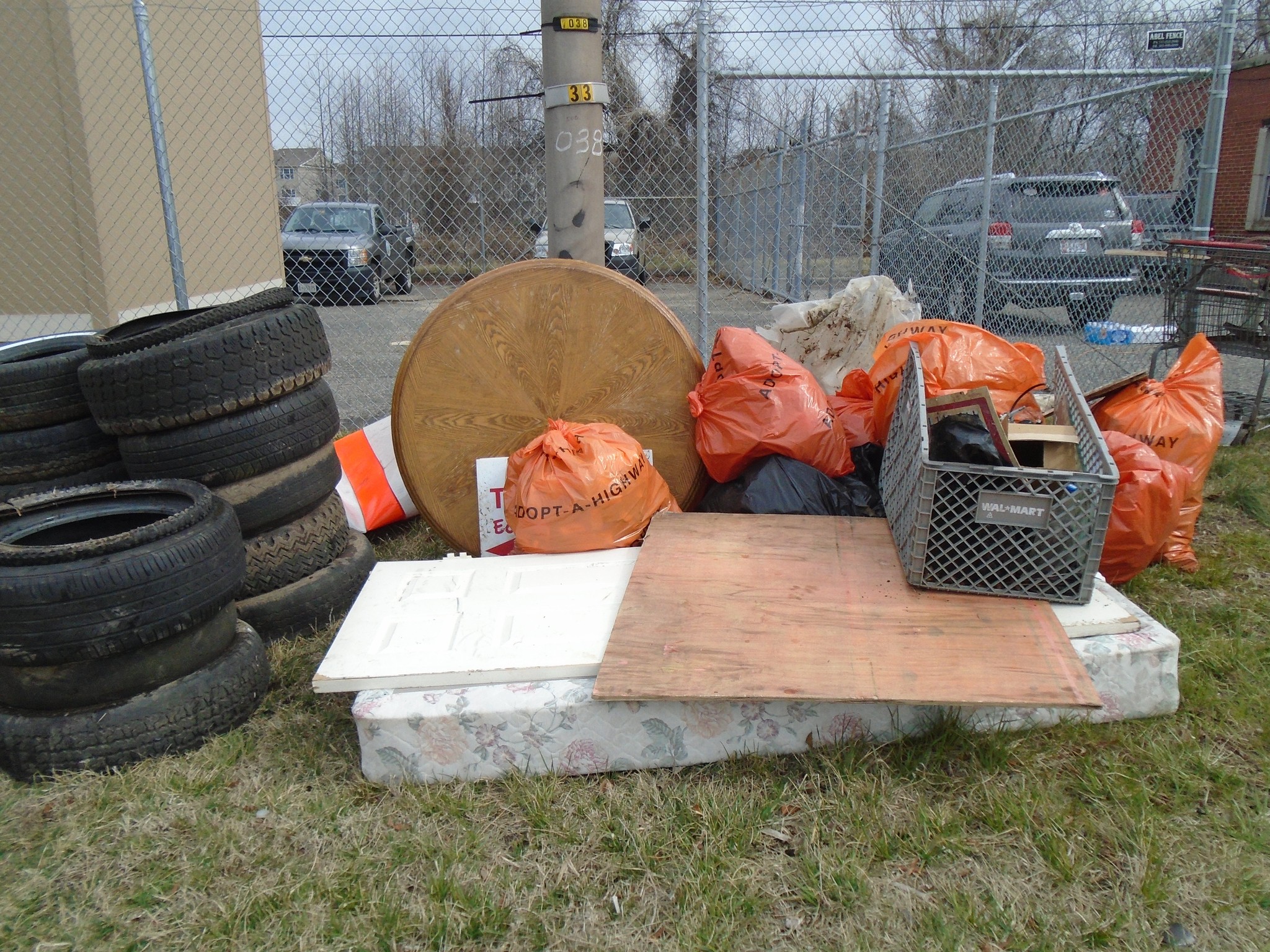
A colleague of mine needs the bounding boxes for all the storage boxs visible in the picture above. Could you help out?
[880,337,1121,605]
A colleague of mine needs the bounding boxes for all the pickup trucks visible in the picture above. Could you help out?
[280,201,416,306]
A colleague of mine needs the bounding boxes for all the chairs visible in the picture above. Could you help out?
[315,213,331,228]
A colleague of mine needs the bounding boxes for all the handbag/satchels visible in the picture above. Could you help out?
[687,323,855,482]
[1090,331,1224,570]
[504,418,683,552]
[1096,430,1193,587]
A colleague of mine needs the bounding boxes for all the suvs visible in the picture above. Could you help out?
[530,199,651,287]
[879,170,1144,331]
[1119,189,1221,297]
